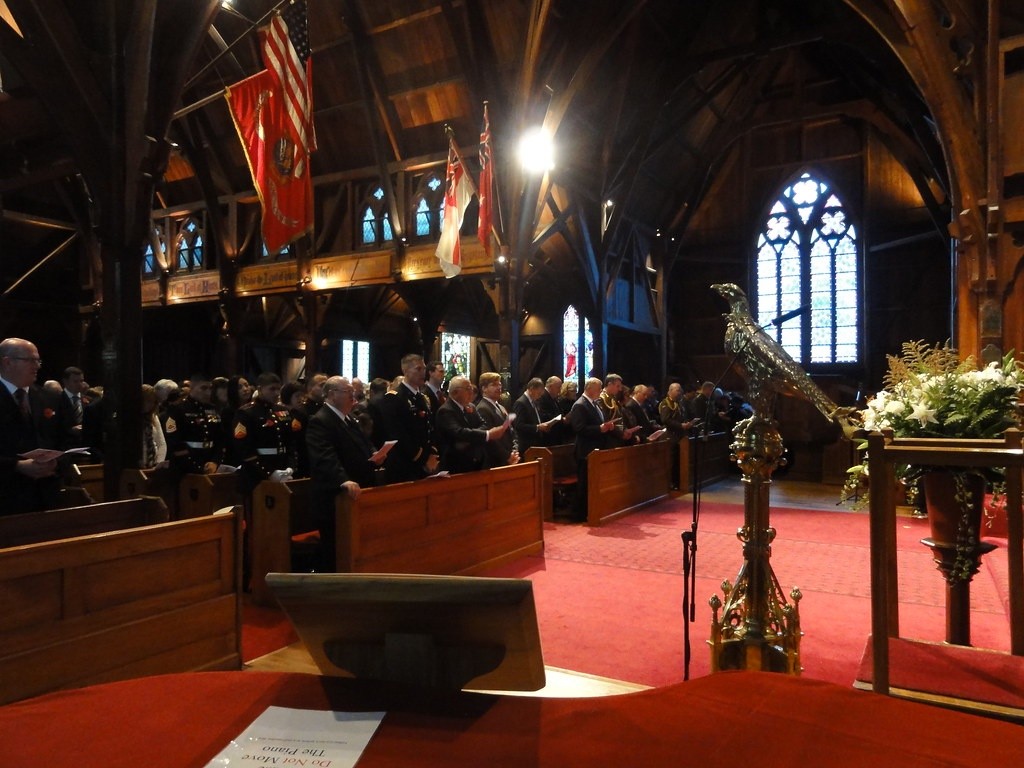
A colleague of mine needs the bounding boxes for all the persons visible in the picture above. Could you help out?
[0,338,756,574]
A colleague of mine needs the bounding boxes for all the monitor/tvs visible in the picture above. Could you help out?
[266,572,546,692]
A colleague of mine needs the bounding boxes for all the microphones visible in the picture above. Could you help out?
[772,304,811,326]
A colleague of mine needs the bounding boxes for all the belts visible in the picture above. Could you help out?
[257,447,286,455]
[186,441,213,449]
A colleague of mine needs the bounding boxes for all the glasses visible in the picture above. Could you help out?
[7,358,42,365]
[456,386,473,391]
[488,384,502,388]
[334,389,355,394]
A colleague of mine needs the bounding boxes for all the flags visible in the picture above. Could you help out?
[434,135,475,279]
[257,0,318,166]
[476,106,493,258]
[225,70,314,261]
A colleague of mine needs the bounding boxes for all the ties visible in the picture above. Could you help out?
[496,403,502,413]
[532,400,536,409]
[464,407,472,426]
[345,417,364,442]
[72,395,83,424]
[593,402,604,422]
[436,391,444,402]
[14,389,31,419]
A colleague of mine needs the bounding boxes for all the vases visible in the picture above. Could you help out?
[924,469,984,546]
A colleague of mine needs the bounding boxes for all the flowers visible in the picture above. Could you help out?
[832,340,1024,511]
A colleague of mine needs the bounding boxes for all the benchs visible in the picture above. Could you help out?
[0,443,580,609]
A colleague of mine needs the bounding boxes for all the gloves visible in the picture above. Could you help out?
[269,468,294,483]
